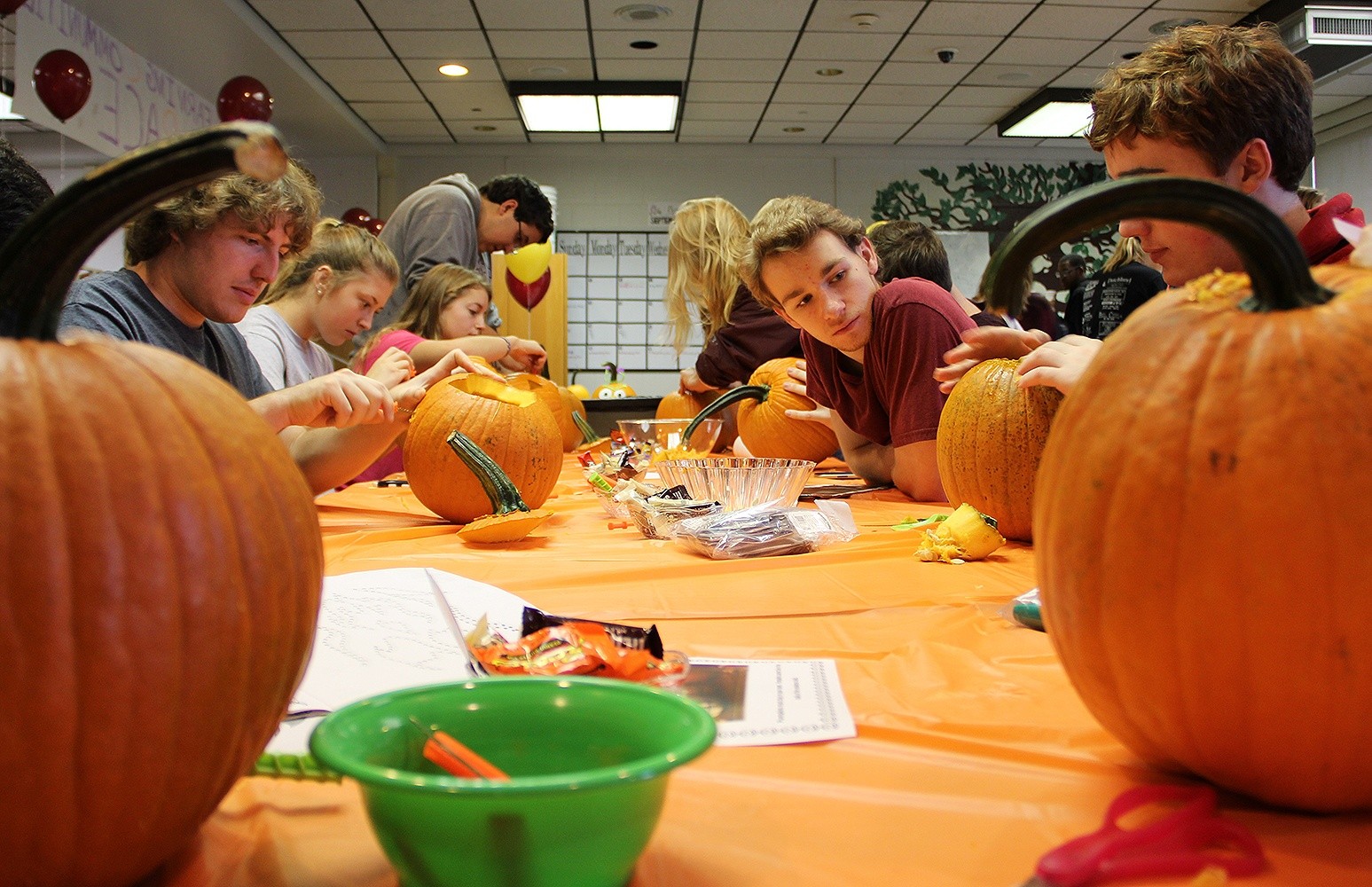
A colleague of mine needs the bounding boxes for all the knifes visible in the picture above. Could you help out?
[424,569,491,677]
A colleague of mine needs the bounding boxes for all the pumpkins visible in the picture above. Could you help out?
[978,176,1372,811]
[570,409,614,453]
[654,387,747,453]
[681,357,841,464]
[403,375,564,541]
[568,371,590,400]
[557,386,587,453]
[3,117,330,887]
[935,358,1079,546]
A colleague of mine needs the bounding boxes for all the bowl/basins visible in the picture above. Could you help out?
[309,675,718,886]
[653,457,817,509]
[616,419,724,457]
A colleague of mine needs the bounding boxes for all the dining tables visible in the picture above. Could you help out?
[176,450,1371,887]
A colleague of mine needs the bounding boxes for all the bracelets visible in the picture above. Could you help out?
[501,337,510,358]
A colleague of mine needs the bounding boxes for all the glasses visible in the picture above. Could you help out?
[510,220,524,255]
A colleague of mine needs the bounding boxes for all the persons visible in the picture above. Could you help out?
[1065,236,1167,343]
[736,196,980,502]
[59,153,506,497]
[661,198,805,395]
[934,23,1366,401]
[1295,185,1328,213]
[782,220,1008,433]
[333,263,548,492]
[1022,294,1056,340]
[1058,254,1090,333]
[233,214,417,391]
[348,171,554,356]
[1,136,59,265]
